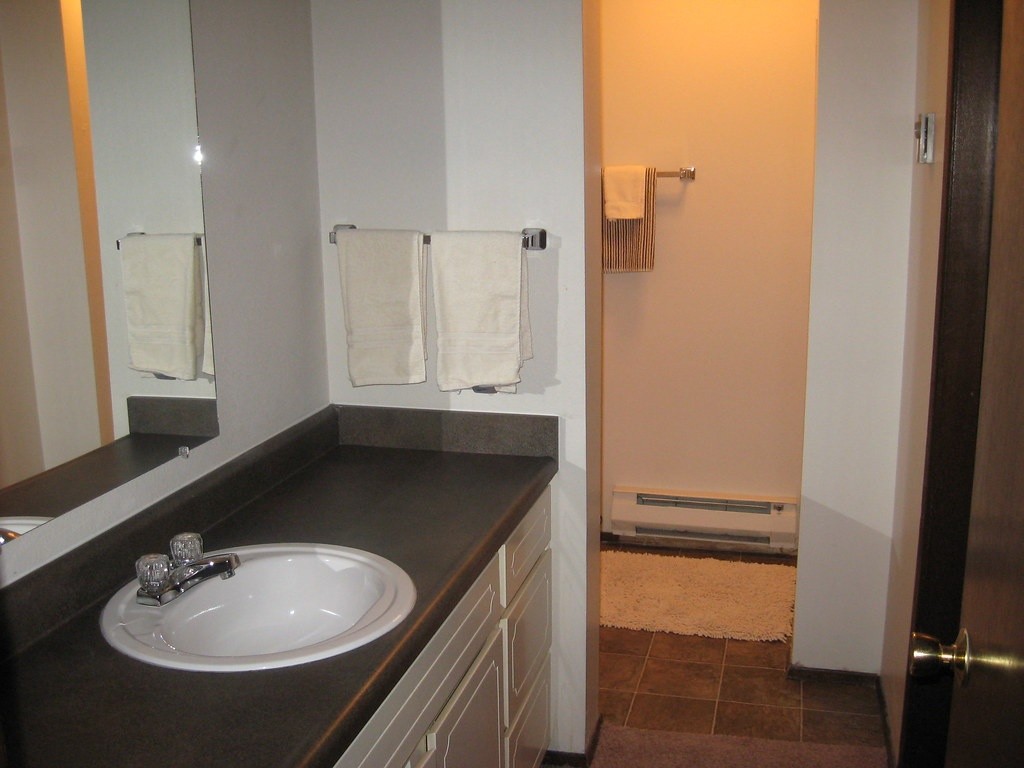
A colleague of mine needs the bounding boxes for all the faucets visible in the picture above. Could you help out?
[135,551,242,607]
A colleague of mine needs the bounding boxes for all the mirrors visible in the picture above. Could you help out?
[0,0,220,553]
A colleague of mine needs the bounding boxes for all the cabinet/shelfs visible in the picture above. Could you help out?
[332,486,554,768]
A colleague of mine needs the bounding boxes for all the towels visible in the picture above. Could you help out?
[334,225,430,387]
[120,232,204,382]
[600,166,658,271]
[432,231,535,396]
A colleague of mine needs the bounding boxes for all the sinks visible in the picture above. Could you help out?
[1,514,53,545]
[99,539,419,674]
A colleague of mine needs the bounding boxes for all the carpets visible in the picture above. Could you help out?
[599,550,799,642]
